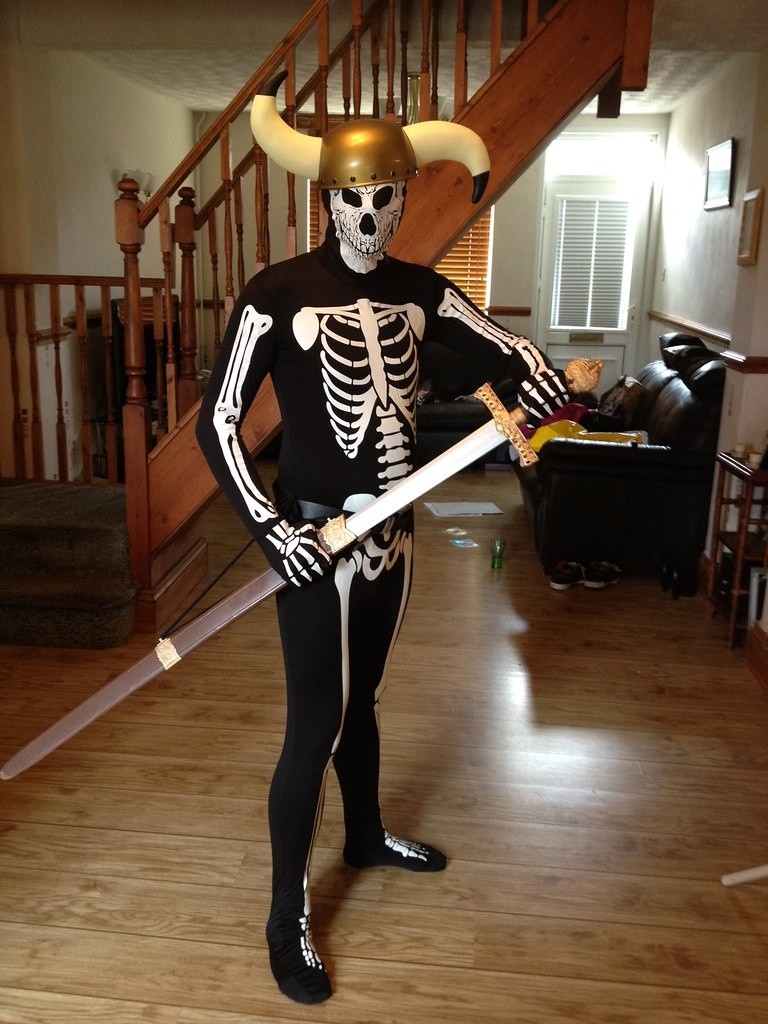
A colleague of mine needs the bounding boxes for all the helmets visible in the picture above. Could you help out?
[247,67,500,202]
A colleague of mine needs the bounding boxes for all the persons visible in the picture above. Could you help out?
[193,70,571,1007]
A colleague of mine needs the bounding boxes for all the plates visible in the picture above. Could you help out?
[730,449,748,457]
[745,461,761,469]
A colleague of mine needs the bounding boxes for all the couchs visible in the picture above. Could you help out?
[519,327,730,583]
[414,380,523,473]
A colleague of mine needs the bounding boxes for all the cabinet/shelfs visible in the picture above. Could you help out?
[703,444,768,656]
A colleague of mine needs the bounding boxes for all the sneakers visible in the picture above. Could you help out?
[549,560,585,591]
[585,560,622,588]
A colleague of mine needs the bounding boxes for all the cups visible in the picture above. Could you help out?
[490,538,504,569]
[750,452,762,468]
[734,443,746,456]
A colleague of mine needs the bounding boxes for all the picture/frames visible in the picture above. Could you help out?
[703,136,735,212]
[736,187,766,268]
